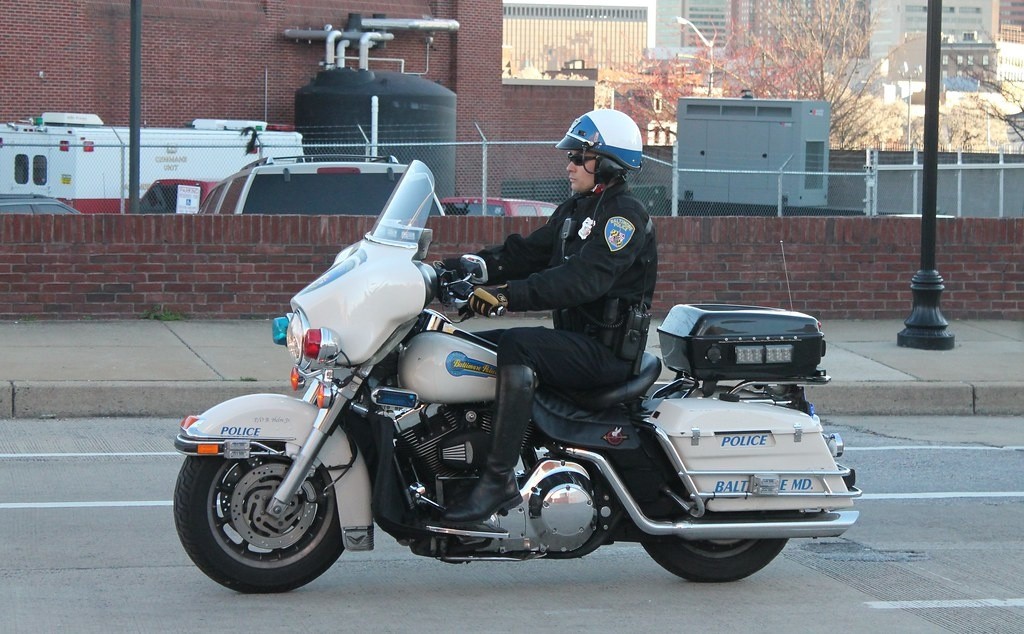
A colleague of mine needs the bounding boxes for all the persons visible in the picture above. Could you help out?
[437,110,658,521]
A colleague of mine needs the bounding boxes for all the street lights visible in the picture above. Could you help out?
[677,17,713,97]
[902,69,917,151]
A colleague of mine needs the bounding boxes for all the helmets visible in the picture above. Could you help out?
[555,109,643,171]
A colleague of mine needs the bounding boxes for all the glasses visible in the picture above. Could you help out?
[567,152,597,166]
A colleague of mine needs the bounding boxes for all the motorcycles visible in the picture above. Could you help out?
[173,159,864,594]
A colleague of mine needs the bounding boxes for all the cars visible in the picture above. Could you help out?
[140,179,217,214]
[0,194,81,214]
[197,156,446,216]
[439,197,558,216]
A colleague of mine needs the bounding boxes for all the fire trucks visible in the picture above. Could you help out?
[0,112,305,214]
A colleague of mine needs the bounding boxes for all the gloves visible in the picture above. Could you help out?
[468,284,509,319]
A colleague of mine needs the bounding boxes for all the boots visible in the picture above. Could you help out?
[442,365,536,528]
